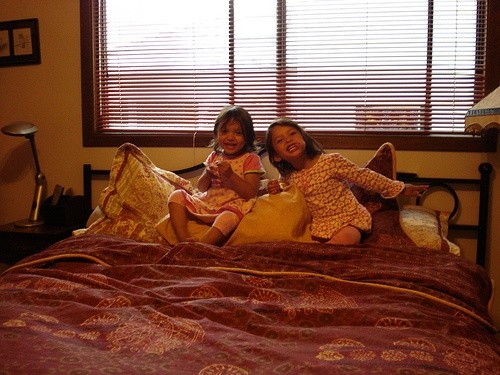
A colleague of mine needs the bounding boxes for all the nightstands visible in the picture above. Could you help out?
[0,219,71,236]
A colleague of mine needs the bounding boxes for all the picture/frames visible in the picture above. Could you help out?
[0,18,41,68]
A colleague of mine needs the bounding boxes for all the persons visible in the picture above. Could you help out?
[168,106,266,246]
[264,118,429,244]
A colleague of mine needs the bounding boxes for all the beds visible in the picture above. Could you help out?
[0,143,500,375]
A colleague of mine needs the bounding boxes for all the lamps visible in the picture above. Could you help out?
[465,86,500,136]
[0,122,46,228]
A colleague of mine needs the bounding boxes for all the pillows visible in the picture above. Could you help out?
[72,142,462,258]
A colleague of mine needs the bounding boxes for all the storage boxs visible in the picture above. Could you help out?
[42,195,72,228]
